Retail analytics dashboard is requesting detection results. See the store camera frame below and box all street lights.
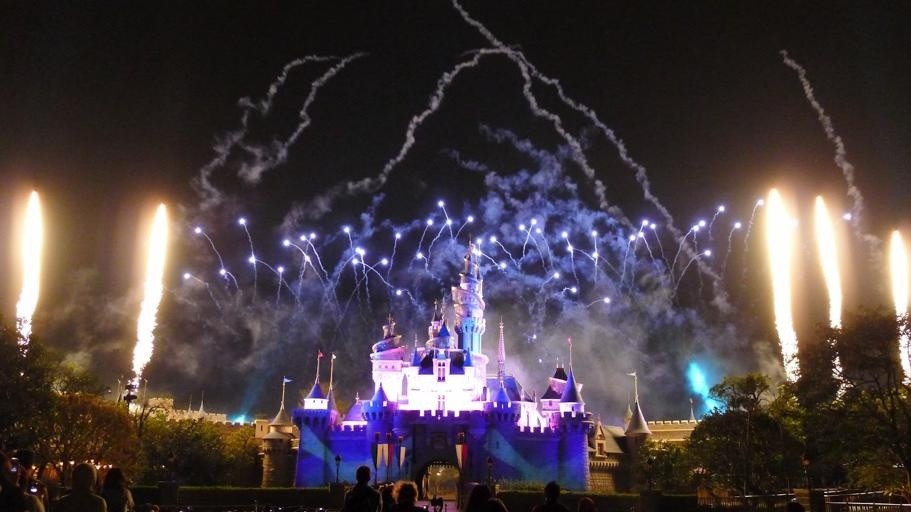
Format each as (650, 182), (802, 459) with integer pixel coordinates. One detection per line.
(334, 453), (341, 483)
(487, 456), (493, 483)
(454, 431), (469, 471)
(383, 432), (395, 482)
(372, 432), (382, 487)
(396, 436), (406, 479)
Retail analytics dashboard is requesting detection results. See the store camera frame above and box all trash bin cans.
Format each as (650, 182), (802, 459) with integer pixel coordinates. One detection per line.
(156, 481), (179, 506)
(809, 489), (826, 512)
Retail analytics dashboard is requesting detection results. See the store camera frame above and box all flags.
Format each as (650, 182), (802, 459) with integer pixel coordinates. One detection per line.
(318, 352), (323, 358)
(332, 353), (336, 360)
(284, 378), (293, 383)
(626, 372), (637, 377)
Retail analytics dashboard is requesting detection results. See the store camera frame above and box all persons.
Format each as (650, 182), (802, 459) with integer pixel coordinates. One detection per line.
(0, 448), (160, 512)
(337, 463), (596, 512)
